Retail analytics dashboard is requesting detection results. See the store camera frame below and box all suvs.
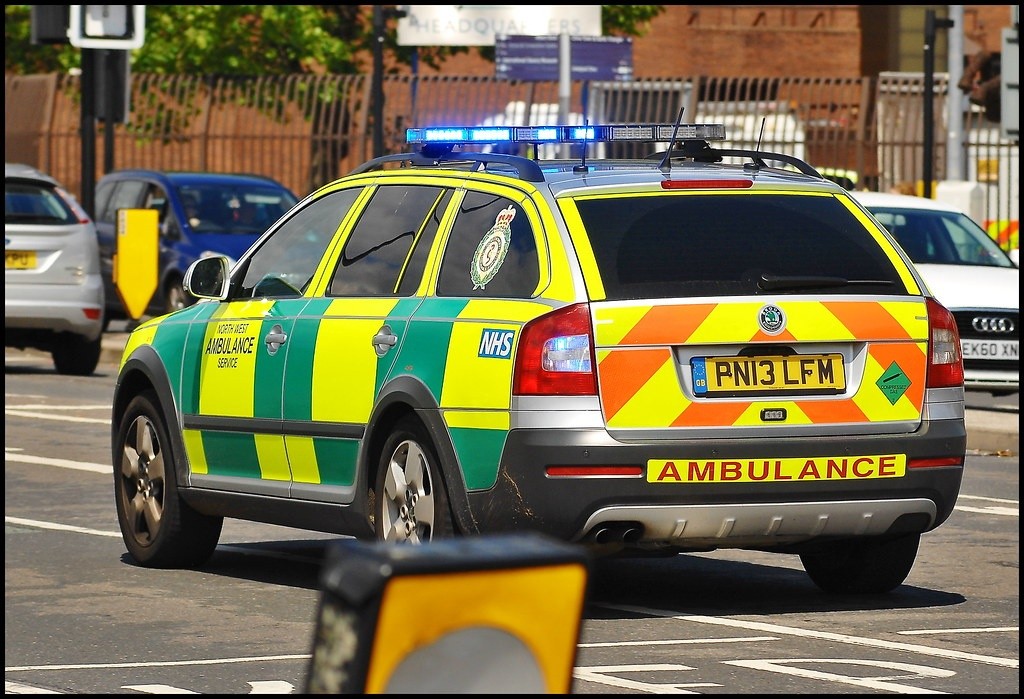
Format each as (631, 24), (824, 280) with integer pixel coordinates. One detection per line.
(111, 123), (968, 598)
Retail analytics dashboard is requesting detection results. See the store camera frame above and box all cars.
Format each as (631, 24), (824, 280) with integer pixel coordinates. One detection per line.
(843, 188), (1019, 401)
(4, 160), (108, 378)
(92, 168), (328, 335)
